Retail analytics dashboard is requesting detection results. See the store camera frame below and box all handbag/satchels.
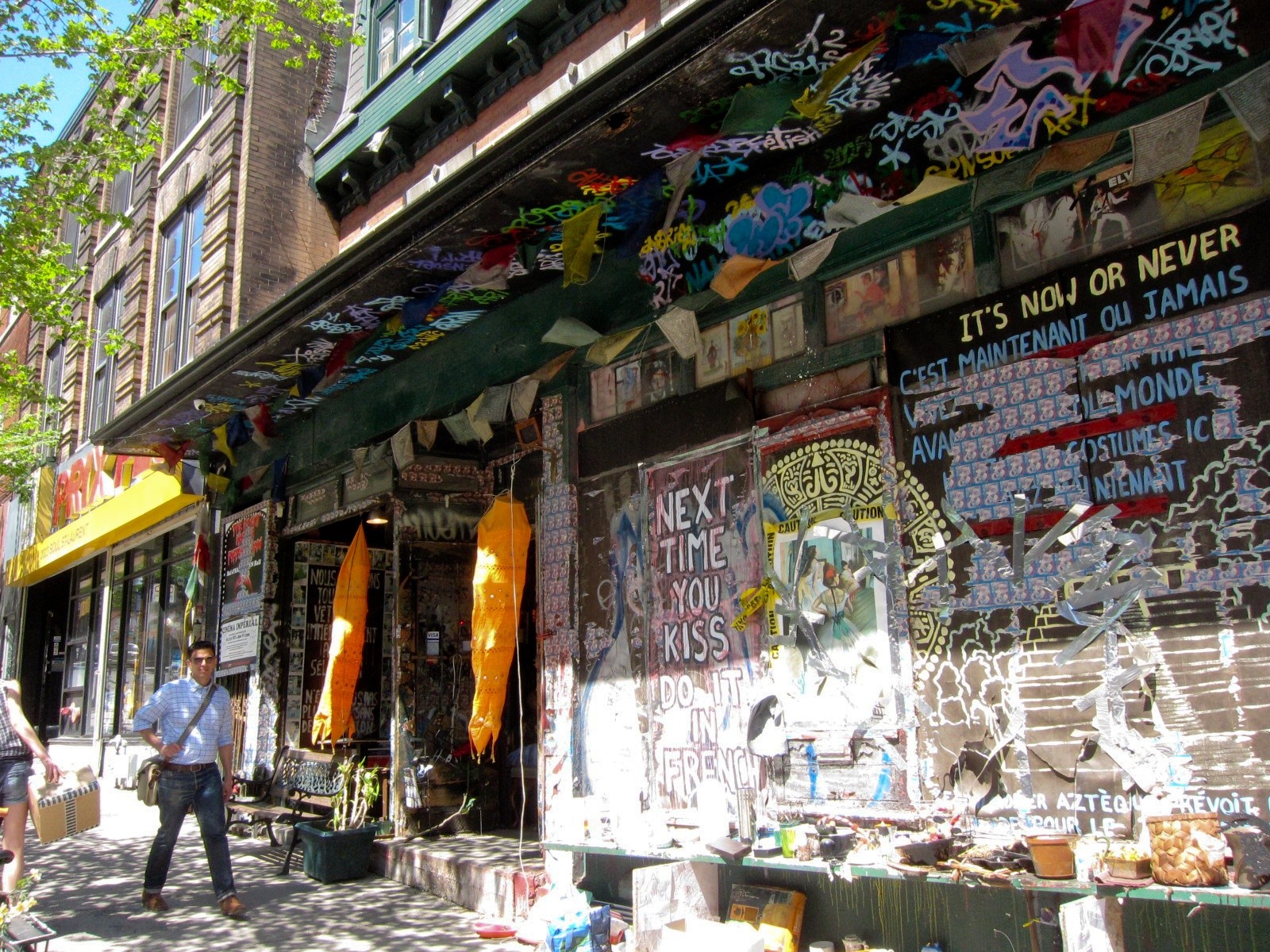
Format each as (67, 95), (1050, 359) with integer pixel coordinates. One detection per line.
(138, 757), (163, 806)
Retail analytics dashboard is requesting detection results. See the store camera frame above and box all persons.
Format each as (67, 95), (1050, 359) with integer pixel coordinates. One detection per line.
(0, 677), (68, 908)
(133, 641), (250, 919)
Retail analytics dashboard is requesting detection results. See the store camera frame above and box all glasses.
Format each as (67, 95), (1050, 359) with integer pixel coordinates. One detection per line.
(189, 657), (215, 663)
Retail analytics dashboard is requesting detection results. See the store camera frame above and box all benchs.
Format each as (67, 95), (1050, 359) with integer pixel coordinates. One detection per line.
(539, 832), (1269, 951)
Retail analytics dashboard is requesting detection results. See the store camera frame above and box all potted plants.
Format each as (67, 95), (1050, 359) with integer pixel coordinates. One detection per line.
(293, 755), (381, 885)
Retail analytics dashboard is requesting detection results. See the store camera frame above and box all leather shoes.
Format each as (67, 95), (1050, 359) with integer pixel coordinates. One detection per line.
(142, 890), (168, 911)
(219, 896), (247, 916)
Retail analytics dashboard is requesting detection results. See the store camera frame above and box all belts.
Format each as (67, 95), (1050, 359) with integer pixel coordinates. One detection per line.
(158, 761), (217, 772)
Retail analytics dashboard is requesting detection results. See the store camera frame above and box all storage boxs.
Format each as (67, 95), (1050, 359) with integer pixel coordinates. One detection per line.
(28, 763), (102, 846)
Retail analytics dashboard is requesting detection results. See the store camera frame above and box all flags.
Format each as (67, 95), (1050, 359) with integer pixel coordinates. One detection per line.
(151, 0), (1125, 666)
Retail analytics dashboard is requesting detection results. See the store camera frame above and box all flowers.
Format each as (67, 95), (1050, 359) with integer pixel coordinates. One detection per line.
(0, 867), (43, 944)
